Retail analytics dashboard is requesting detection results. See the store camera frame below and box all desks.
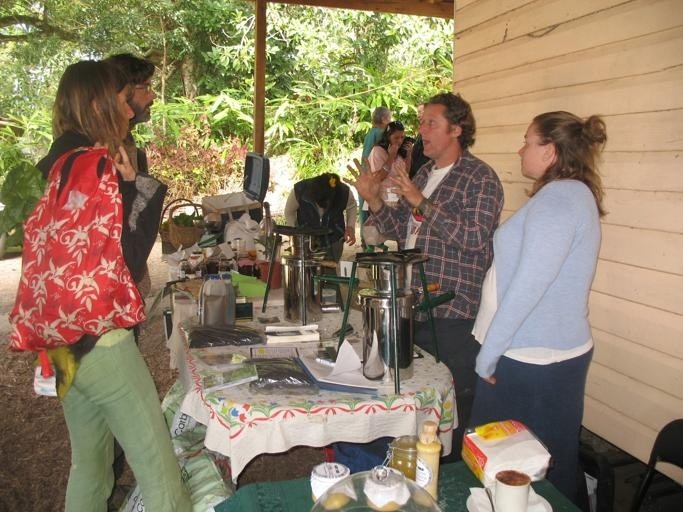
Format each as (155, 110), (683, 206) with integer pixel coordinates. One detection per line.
(176, 294), (458, 477)
(215, 462), (577, 510)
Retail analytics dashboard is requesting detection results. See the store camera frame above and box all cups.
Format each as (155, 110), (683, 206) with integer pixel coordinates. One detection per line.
(494, 467), (532, 511)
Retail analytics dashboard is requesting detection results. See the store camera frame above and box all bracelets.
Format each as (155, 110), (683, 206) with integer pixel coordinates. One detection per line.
(381, 162), (393, 175)
(416, 198), (427, 215)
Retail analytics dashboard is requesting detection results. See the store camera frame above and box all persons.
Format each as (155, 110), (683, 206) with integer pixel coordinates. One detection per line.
(407, 102), (432, 180)
(97, 52), (148, 480)
(359, 119), (412, 253)
(340, 93), (507, 461)
(6, 61), (185, 512)
(359, 107), (392, 226)
(285, 172), (357, 271)
(459, 111), (608, 512)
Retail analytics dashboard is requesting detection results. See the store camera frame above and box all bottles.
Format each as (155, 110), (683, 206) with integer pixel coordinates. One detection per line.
(241, 251), (262, 279)
(415, 422), (442, 509)
(389, 434), (416, 480)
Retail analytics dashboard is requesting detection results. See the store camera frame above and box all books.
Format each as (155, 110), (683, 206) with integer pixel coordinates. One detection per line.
(202, 364), (258, 393)
(299, 340), (378, 390)
(293, 357), (376, 394)
(264, 324), (319, 345)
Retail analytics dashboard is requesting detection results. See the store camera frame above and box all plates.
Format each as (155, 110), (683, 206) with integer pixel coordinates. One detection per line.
(464, 486), (553, 511)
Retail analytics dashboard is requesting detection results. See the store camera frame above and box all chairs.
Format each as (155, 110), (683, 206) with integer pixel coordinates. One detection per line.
(627, 417), (683, 512)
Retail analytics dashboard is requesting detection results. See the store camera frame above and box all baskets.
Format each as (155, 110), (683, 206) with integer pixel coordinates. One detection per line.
(158, 197), (209, 255)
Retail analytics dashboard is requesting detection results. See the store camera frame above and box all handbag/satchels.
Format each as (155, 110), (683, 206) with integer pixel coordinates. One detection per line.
(6, 147), (149, 352)
